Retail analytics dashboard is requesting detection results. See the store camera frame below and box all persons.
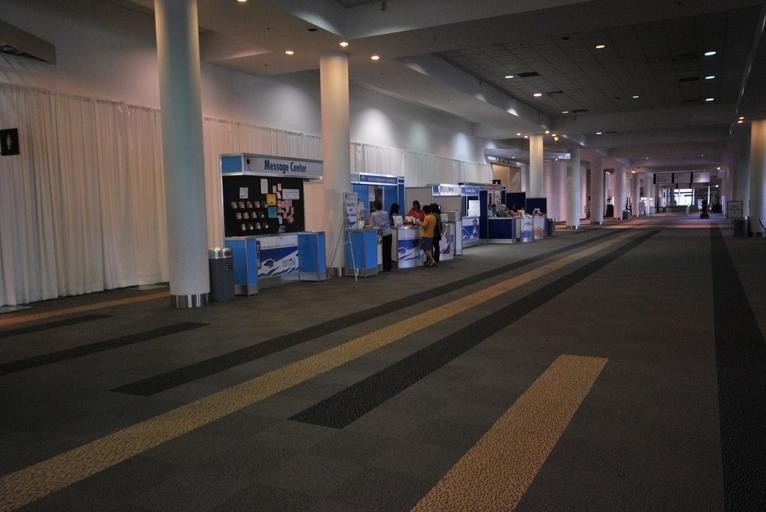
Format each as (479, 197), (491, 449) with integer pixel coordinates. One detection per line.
(369, 200), (443, 273)
(701, 197), (707, 214)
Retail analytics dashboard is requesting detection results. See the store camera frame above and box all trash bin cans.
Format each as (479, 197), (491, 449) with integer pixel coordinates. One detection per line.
(734, 215), (749, 236)
(623, 209), (630, 220)
(208, 247), (236, 303)
(547, 217), (555, 237)
